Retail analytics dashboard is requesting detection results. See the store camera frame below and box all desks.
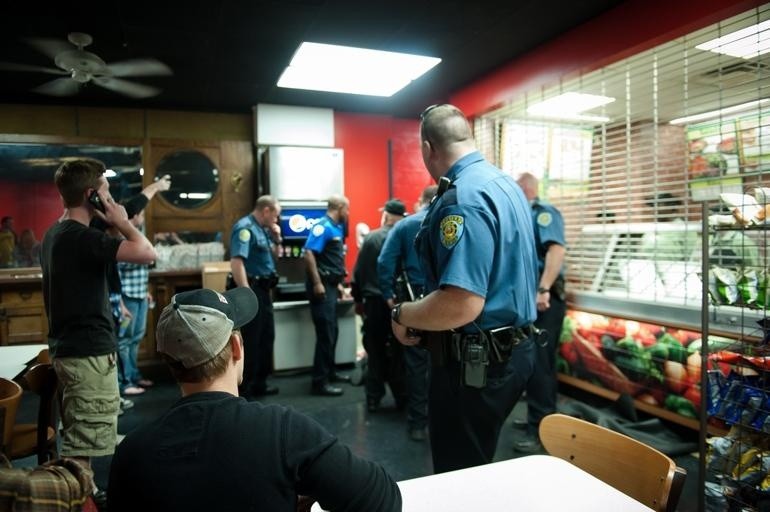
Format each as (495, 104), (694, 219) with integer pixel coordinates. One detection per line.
(0, 344), (49, 382)
(311, 454), (658, 512)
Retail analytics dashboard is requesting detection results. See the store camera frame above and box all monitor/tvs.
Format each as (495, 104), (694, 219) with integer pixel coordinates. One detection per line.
(276, 202), (327, 239)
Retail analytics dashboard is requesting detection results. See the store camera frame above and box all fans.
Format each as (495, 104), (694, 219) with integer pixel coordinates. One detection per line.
(3, 32), (172, 100)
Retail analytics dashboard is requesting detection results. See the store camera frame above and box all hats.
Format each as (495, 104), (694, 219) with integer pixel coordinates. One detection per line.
(378, 199), (407, 218)
(156, 286), (259, 376)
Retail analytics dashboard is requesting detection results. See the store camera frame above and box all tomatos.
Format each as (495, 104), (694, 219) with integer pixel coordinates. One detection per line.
(560, 309), (762, 427)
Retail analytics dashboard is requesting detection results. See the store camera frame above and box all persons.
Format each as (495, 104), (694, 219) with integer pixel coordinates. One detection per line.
(637, 192), (706, 263)
(352, 172), (570, 456)
(39, 157), (173, 499)
(303, 192), (351, 396)
(593, 208), (617, 224)
(0, 455), (95, 512)
(0, 216), (42, 268)
(224, 194), (284, 404)
(106, 287), (403, 511)
(389, 101), (537, 476)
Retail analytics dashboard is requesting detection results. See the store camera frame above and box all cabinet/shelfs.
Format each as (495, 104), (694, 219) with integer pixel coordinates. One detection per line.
(693, 185), (770, 512)
(558, 223), (770, 436)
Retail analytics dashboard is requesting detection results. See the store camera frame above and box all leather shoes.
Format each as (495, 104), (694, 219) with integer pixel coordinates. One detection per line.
(512, 434), (544, 454)
(513, 418), (529, 431)
(366, 399), (379, 410)
(251, 385), (278, 397)
(410, 423), (427, 441)
(309, 383), (344, 396)
(327, 372), (351, 383)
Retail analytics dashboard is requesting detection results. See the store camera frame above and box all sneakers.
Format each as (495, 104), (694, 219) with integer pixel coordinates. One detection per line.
(136, 379), (153, 386)
(118, 397), (134, 408)
(121, 386), (145, 394)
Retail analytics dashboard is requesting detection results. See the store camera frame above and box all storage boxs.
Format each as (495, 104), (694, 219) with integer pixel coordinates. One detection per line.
(201, 261), (232, 292)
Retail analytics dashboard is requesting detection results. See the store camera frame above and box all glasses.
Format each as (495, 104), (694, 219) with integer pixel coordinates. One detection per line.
(420, 104), (440, 152)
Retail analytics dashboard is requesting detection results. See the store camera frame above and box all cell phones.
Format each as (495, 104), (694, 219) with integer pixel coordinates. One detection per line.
(89, 190), (105, 214)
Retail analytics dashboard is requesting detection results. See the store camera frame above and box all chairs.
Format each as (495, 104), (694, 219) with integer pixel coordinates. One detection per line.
(538, 412), (687, 512)
(0, 349), (61, 466)
